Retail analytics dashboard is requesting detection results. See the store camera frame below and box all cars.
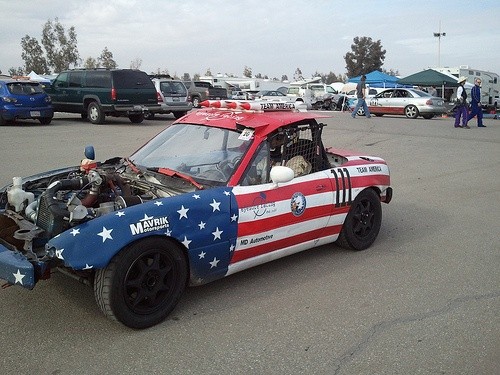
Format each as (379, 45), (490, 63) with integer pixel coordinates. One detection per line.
(350, 88), (449, 119)
(0, 76), (55, 125)
(232, 84), (384, 111)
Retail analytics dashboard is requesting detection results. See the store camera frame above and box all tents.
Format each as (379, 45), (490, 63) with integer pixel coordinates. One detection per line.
(397, 69), (458, 87)
(349, 70), (399, 82)
(17, 71), (51, 84)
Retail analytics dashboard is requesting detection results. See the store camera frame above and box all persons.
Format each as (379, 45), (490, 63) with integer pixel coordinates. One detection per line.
(242, 124), (312, 189)
(453, 77), (471, 129)
(467, 78), (486, 127)
(351, 75), (371, 118)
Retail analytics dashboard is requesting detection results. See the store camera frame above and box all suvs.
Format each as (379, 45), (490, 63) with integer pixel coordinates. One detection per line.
(143, 74), (193, 120)
(41, 67), (161, 124)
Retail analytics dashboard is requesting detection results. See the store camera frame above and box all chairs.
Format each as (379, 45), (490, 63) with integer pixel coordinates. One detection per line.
(13, 85), (22, 93)
(23, 86), (31, 93)
(175, 84), (182, 91)
(281, 139), (324, 176)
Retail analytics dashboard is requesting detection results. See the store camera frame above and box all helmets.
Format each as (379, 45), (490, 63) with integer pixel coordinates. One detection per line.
(267, 124), (300, 158)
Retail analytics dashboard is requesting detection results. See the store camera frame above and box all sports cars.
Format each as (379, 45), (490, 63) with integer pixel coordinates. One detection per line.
(0, 98), (393, 331)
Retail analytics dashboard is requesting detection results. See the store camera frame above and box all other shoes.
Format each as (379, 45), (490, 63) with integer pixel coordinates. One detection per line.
(366, 116), (371, 118)
(351, 115), (356, 118)
(455, 125), (463, 128)
(462, 125), (470, 129)
(477, 124), (486, 127)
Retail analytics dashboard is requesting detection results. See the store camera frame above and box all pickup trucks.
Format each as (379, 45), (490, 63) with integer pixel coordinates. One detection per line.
(185, 81), (228, 109)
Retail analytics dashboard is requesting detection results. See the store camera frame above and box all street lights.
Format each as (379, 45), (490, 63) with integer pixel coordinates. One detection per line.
(433, 23), (447, 67)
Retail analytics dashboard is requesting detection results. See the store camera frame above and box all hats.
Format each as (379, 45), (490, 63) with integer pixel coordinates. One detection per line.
(457, 76), (468, 83)
